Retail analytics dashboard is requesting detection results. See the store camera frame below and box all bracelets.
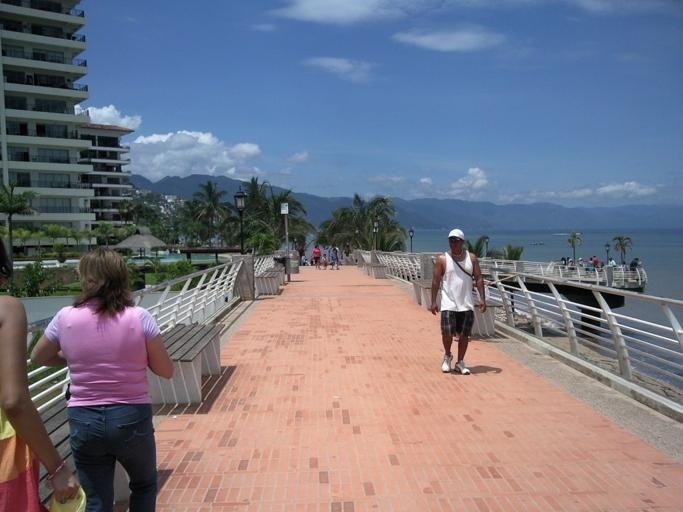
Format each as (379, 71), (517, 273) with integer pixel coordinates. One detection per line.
(46, 460), (66, 479)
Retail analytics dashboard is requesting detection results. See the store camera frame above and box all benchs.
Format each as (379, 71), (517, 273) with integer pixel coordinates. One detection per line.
(254, 270), (282, 296)
(364, 261), (388, 279)
(263, 265), (287, 287)
(148, 321), (226, 405)
(470, 295), (504, 339)
(412, 278), (442, 314)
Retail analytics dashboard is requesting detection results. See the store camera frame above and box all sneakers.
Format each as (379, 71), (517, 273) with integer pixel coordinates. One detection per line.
(455, 361), (470, 375)
(440, 354), (452, 372)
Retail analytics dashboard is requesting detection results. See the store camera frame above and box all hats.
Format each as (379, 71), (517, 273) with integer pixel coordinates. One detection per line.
(448, 228), (464, 239)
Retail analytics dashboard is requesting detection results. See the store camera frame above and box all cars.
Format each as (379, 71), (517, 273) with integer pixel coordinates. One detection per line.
(11, 258), (82, 271)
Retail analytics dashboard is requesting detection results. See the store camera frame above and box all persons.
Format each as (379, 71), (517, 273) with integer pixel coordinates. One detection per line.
(561, 255), (640, 271)
(301, 242), (351, 270)
(430, 229), (486, 375)
(31, 247), (175, 512)
(0, 239), (79, 512)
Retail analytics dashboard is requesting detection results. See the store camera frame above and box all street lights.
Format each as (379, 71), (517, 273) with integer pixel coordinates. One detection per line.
(293, 236), (296, 249)
(408, 225), (414, 253)
(484, 236), (490, 253)
(234, 184), (247, 255)
(604, 241), (611, 265)
(371, 215), (379, 250)
(354, 228), (360, 249)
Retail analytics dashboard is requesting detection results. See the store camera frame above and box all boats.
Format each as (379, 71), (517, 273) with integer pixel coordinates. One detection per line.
(528, 241), (544, 245)
(551, 232), (582, 236)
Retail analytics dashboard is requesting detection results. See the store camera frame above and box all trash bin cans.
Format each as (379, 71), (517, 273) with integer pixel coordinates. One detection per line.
(273, 257), (287, 275)
